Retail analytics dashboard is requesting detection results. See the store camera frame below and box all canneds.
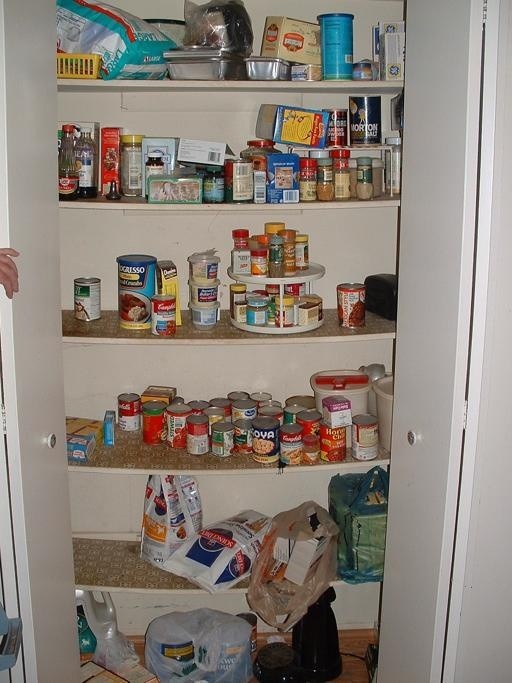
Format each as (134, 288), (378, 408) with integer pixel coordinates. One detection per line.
(116, 254), (156, 327)
(151, 295), (176, 338)
(118, 392), (380, 467)
(337, 282), (367, 329)
(348, 93), (382, 147)
(224, 158), (254, 203)
(230, 282), (306, 328)
(322, 108), (347, 148)
(74, 277), (101, 321)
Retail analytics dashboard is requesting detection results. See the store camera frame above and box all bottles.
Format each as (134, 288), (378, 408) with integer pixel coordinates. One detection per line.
(56, 122), (95, 200)
(119, 132), (144, 199)
(229, 221), (312, 278)
(241, 139), (279, 161)
(299, 148), (384, 200)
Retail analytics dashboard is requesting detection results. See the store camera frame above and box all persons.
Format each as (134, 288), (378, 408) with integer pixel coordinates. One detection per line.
(0, 247), (21, 300)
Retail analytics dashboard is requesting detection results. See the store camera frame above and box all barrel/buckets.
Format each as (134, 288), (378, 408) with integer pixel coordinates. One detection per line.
(308, 369), (372, 418)
(76, 590), (119, 665)
(372, 375), (394, 454)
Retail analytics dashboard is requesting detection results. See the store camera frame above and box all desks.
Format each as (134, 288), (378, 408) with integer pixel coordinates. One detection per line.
(80, 630), (377, 680)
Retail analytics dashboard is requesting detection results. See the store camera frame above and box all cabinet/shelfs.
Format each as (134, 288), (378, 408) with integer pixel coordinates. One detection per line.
(49, 62), (395, 606)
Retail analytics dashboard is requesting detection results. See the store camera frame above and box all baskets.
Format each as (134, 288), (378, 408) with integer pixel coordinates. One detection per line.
(56, 53), (102, 78)
